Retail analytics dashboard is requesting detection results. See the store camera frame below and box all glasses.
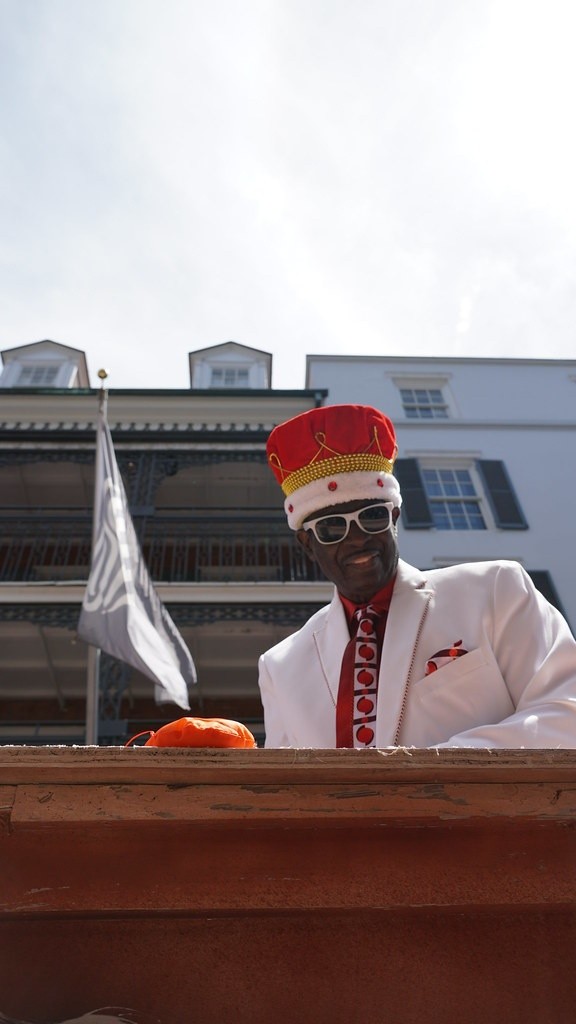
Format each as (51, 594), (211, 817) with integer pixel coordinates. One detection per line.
(303, 502), (392, 545)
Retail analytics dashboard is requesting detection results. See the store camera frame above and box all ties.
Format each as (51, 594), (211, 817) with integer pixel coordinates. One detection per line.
(336, 607), (381, 748)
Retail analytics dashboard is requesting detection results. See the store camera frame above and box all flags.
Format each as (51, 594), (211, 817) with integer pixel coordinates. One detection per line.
(75, 411), (200, 712)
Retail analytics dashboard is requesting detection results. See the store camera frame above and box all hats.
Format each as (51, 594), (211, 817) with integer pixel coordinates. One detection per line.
(265, 404), (402, 531)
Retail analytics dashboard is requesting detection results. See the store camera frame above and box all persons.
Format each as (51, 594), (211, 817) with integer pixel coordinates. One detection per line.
(255, 404), (576, 752)
